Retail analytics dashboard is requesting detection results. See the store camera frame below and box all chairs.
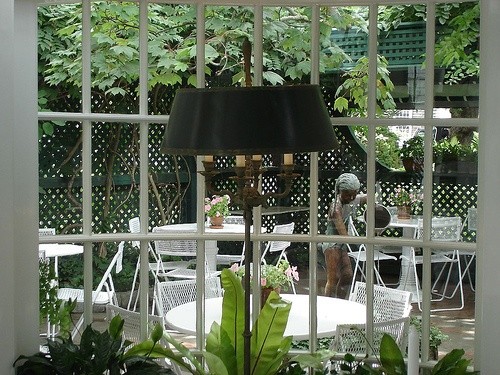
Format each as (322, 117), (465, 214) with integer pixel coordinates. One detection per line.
(105, 277), (413, 370)
(127, 217), (296, 311)
(39, 228), (124, 342)
(346, 208), (477, 313)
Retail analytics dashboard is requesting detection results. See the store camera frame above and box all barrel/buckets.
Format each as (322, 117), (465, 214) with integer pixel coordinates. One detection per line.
(364, 204), (393, 235)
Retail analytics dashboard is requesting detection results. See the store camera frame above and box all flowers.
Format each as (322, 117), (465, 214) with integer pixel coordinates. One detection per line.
(390, 188), (417, 206)
(205, 195), (231, 217)
(230, 258), (299, 291)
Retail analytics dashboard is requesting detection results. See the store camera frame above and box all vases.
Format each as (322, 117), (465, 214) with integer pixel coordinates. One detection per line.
(263, 289), (280, 308)
(397, 204), (411, 219)
(211, 216), (225, 226)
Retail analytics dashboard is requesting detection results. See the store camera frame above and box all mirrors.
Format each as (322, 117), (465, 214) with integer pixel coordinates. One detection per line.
(364, 204), (392, 229)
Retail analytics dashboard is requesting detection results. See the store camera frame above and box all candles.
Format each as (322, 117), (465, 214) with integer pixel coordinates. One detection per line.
(284, 154), (293, 165)
(236, 155), (245, 167)
(204, 155), (213, 162)
(253, 155), (262, 160)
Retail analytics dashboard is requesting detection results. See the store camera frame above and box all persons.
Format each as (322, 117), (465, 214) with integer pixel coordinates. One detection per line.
(322, 173), (367, 299)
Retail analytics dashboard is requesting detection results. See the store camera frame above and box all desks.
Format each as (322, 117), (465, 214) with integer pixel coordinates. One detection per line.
(166, 293), (367, 341)
(38, 244), (84, 339)
(156, 223), (267, 271)
(229, 206), (309, 215)
(356, 215), (457, 304)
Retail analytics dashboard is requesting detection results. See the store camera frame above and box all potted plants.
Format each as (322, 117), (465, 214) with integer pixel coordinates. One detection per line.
(414, 318), (449, 361)
(394, 135), (478, 174)
